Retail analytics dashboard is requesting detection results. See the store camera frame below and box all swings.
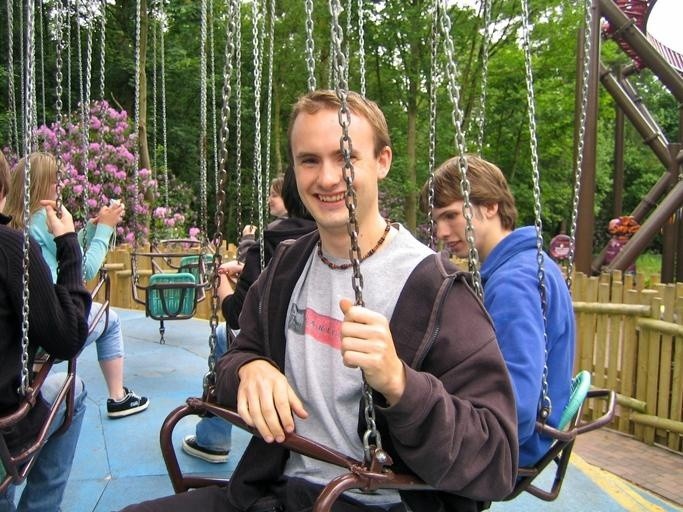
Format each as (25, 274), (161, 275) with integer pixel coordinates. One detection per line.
(0, 3), (615, 512)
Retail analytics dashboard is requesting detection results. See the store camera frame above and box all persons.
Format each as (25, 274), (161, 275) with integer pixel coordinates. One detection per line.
(234, 177), (288, 278)
(602, 214), (640, 286)
(181, 164), (318, 466)
(3, 150), (149, 419)
(0, 149), (87, 510)
(115, 88), (519, 510)
(416, 151), (576, 490)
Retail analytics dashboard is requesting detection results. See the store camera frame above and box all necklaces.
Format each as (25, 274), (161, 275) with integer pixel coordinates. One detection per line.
(314, 220), (393, 270)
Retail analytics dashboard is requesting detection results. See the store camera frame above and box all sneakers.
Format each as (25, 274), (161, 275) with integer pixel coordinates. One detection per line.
(182, 435), (229, 464)
(107, 387), (149, 417)
